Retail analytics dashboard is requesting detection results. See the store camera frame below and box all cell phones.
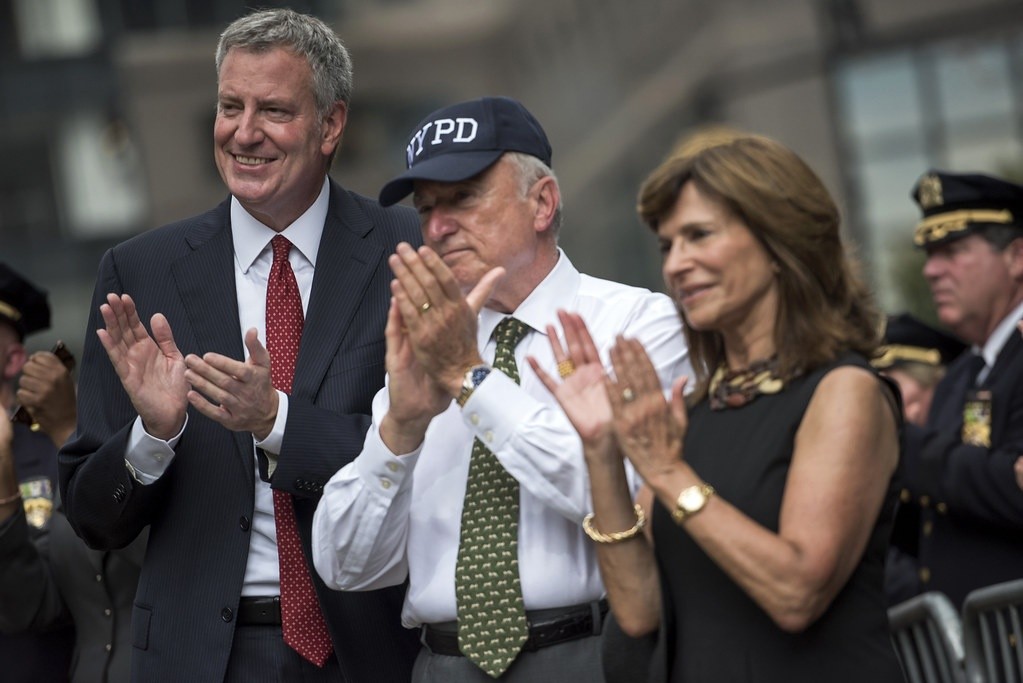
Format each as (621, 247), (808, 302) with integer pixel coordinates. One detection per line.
(10, 340), (75, 428)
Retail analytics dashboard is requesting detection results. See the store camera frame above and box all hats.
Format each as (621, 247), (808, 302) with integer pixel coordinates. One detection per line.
(870, 311), (968, 371)
(379, 98), (552, 209)
(910, 169), (1023, 245)
(0, 264), (52, 331)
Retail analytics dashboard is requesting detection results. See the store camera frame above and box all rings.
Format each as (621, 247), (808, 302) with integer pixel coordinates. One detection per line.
(419, 302), (431, 314)
(621, 386), (635, 403)
(558, 359), (574, 378)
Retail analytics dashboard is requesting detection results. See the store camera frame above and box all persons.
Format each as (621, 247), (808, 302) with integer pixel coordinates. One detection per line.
(311, 96), (697, 683)
(0, 260), (152, 683)
(869, 168), (1023, 683)
(524, 133), (906, 683)
(57, 8), (424, 683)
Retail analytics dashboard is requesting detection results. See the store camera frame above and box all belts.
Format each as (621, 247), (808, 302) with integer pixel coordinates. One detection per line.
(423, 601), (608, 656)
(239, 597), (282, 627)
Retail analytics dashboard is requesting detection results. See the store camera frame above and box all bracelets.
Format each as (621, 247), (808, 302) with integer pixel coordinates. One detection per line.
(0, 489), (23, 504)
(582, 504), (647, 543)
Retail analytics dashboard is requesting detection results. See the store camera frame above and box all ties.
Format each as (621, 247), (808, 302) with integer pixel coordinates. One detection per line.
(270, 235), (333, 666)
(455, 319), (529, 680)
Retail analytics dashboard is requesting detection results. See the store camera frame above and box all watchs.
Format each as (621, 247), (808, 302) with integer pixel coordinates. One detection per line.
(456, 365), (492, 408)
(671, 484), (716, 526)
